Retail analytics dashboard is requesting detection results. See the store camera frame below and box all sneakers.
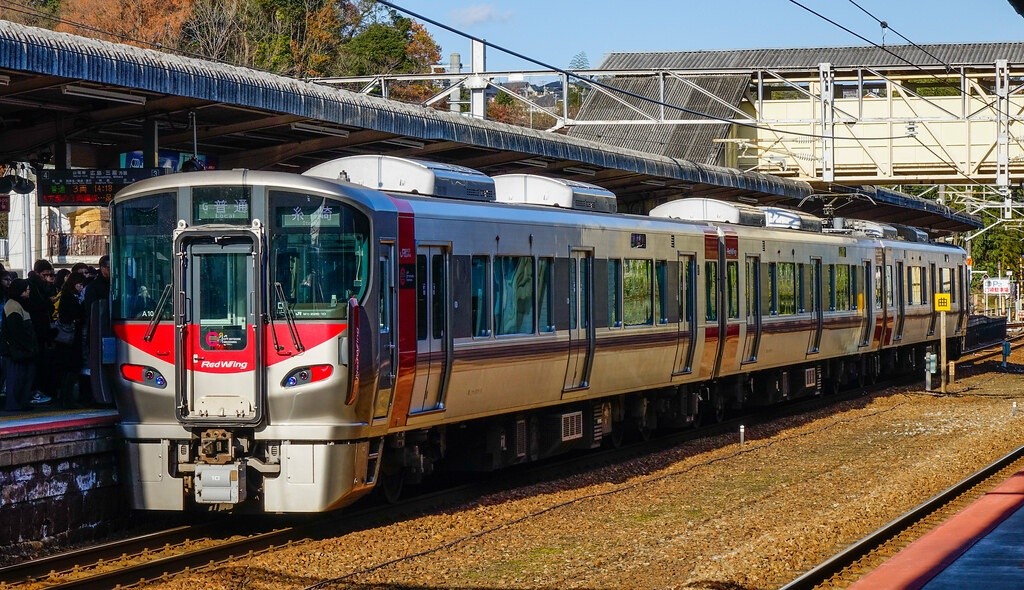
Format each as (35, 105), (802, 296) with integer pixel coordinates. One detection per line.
(29, 391), (52, 403)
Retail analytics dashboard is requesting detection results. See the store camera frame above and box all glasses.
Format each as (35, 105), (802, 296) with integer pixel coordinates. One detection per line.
(42, 273), (54, 277)
(2, 278), (12, 281)
(78, 270), (89, 275)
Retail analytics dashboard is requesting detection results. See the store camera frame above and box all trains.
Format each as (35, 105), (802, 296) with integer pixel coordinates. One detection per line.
(90, 155), (972, 533)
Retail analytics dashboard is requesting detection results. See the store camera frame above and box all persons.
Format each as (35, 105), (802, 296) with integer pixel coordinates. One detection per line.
(1, 255), (158, 412)
